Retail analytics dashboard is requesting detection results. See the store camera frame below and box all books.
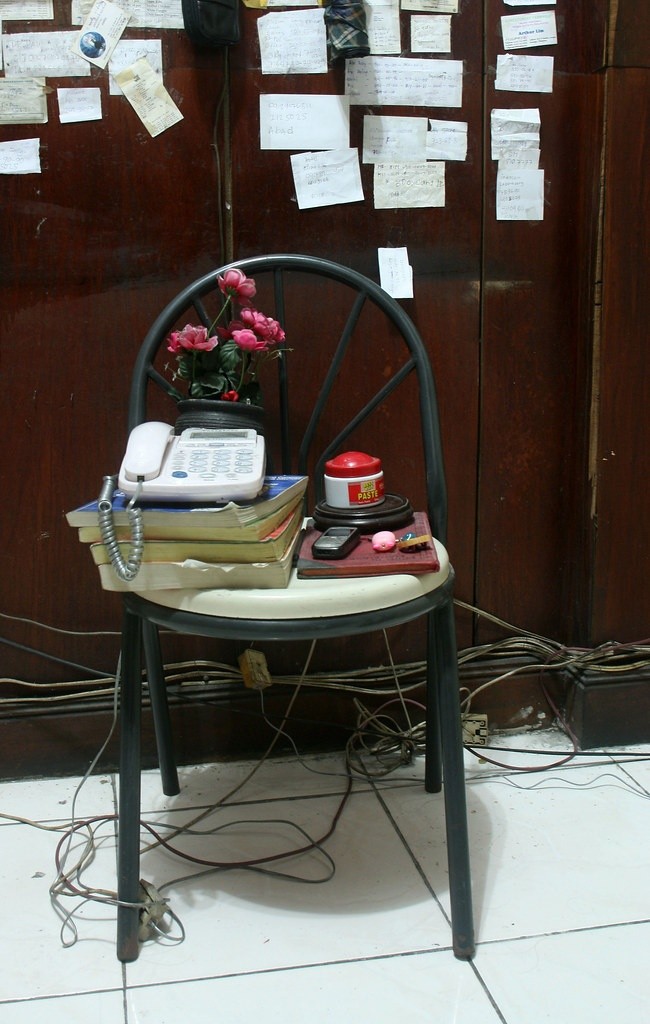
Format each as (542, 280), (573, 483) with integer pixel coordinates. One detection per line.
(296, 512), (441, 579)
(65, 476), (311, 592)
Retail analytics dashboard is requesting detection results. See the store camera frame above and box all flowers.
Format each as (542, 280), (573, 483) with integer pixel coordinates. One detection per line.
(163, 268), (296, 405)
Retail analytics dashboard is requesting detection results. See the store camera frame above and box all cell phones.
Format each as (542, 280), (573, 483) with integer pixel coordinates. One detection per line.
(311, 526), (361, 559)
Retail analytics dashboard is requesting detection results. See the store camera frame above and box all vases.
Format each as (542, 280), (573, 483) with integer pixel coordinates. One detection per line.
(171, 400), (266, 438)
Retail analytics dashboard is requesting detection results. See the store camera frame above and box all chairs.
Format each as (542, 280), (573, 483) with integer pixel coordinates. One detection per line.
(117, 253), (476, 961)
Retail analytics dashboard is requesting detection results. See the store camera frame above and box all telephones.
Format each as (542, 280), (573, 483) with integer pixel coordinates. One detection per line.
(98, 421), (266, 582)
